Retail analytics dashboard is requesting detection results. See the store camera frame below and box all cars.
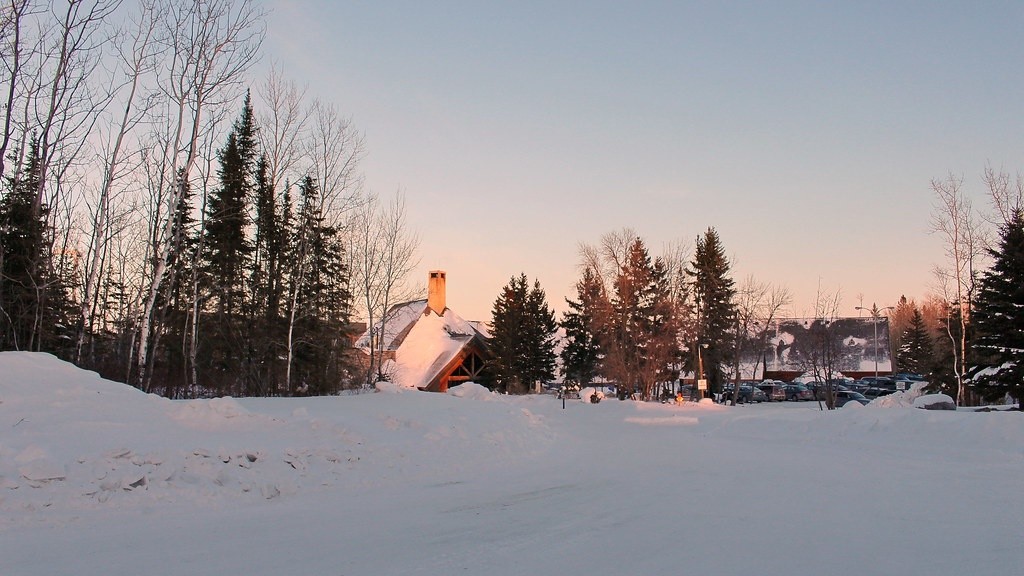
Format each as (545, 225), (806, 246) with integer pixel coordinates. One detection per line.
(825, 390), (872, 408)
(724, 372), (923, 403)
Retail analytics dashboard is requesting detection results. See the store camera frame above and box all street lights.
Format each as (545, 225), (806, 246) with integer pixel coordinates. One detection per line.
(697, 343), (709, 398)
(855, 306), (895, 381)
